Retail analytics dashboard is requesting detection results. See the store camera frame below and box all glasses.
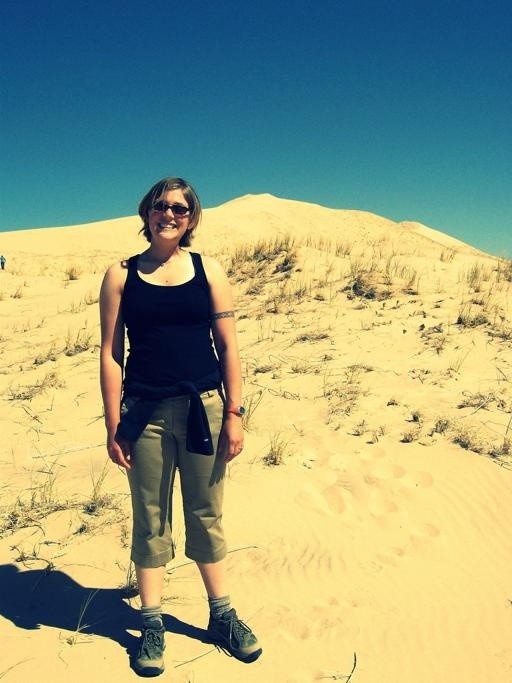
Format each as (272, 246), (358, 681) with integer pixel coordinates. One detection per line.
(152, 200), (192, 214)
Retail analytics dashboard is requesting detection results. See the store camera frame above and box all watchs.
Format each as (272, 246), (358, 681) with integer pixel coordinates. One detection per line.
(225, 405), (245, 415)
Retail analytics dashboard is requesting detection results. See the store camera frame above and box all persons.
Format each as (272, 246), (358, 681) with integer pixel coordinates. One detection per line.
(99, 177), (263, 677)
(0, 255), (6, 270)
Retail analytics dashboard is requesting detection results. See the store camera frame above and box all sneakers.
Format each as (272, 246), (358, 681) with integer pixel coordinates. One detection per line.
(134, 619), (166, 677)
(207, 608), (262, 663)
